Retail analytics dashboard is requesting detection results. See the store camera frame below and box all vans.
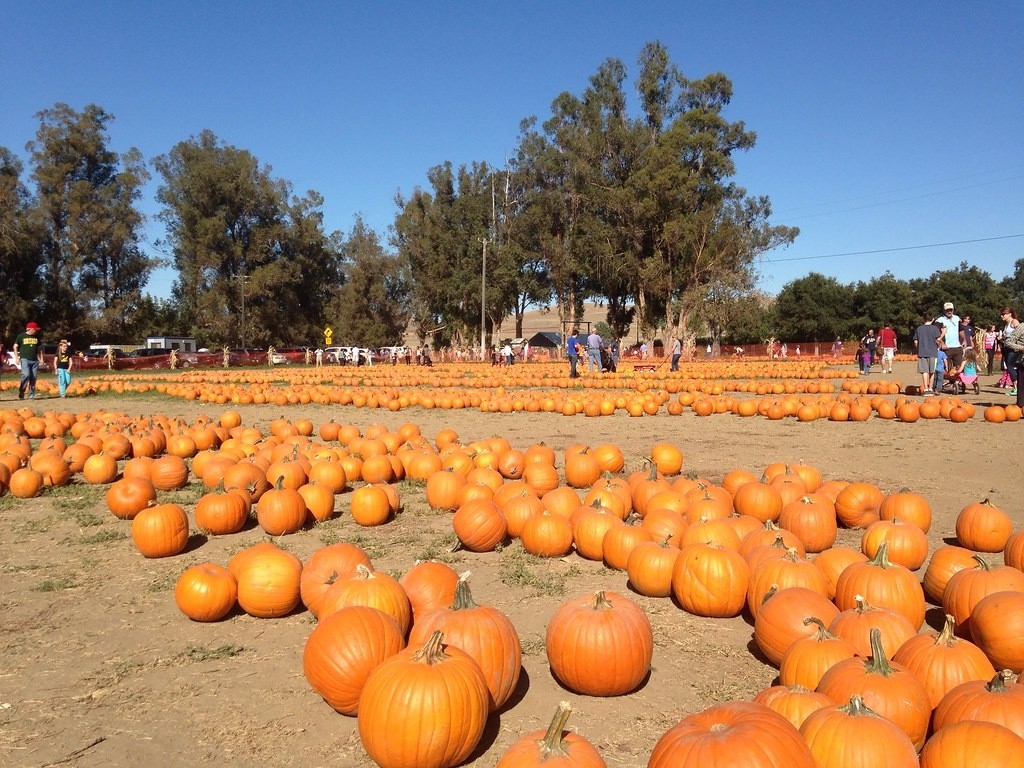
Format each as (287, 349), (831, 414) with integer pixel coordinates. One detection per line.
(305, 347), (406, 363)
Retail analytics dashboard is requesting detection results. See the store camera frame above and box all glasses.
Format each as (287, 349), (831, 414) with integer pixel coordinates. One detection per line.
(1001, 313), (1009, 315)
(672, 337), (674, 338)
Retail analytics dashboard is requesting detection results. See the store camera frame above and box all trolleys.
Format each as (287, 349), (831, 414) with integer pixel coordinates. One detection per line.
(944, 369), (980, 395)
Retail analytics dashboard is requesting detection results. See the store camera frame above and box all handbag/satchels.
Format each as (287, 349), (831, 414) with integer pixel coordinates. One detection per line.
(422, 350), (424, 355)
(1009, 351), (1024, 369)
(875, 345), (883, 356)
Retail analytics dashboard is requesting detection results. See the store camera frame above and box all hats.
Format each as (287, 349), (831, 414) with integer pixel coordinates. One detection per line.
(943, 302), (954, 310)
(27, 322), (41, 330)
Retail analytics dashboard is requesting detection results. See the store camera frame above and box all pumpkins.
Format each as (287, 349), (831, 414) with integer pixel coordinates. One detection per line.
(0, 408), (1024, 768)
(0, 354), (1024, 422)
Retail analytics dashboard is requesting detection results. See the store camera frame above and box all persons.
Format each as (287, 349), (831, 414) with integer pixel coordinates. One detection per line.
(913, 302), (1024, 408)
(640, 342), (647, 360)
(450, 348), (473, 361)
(796, 345), (800, 356)
(856, 329), (877, 375)
(116, 351), (124, 358)
(314, 344), (431, 367)
(13, 322), (43, 399)
(567, 329), (620, 373)
(54, 341), (73, 398)
(79, 350), (84, 357)
(833, 336), (845, 359)
(141, 349), (148, 356)
(733, 346), (744, 359)
(875, 320), (897, 374)
(780, 343), (788, 359)
(670, 337), (681, 372)
(489, 340), (533, 367)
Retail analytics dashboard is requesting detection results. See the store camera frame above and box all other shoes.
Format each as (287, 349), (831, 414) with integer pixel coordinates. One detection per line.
(1005, 389), (1017, 396)
(888, 368), (892, 373)
(923, 391), (934, 396)
(19, 391), (24, 398)
(882, 370), (886, 373)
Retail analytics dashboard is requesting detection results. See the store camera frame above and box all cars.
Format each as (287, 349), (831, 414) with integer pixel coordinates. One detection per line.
(5, 345), (305, 371)
